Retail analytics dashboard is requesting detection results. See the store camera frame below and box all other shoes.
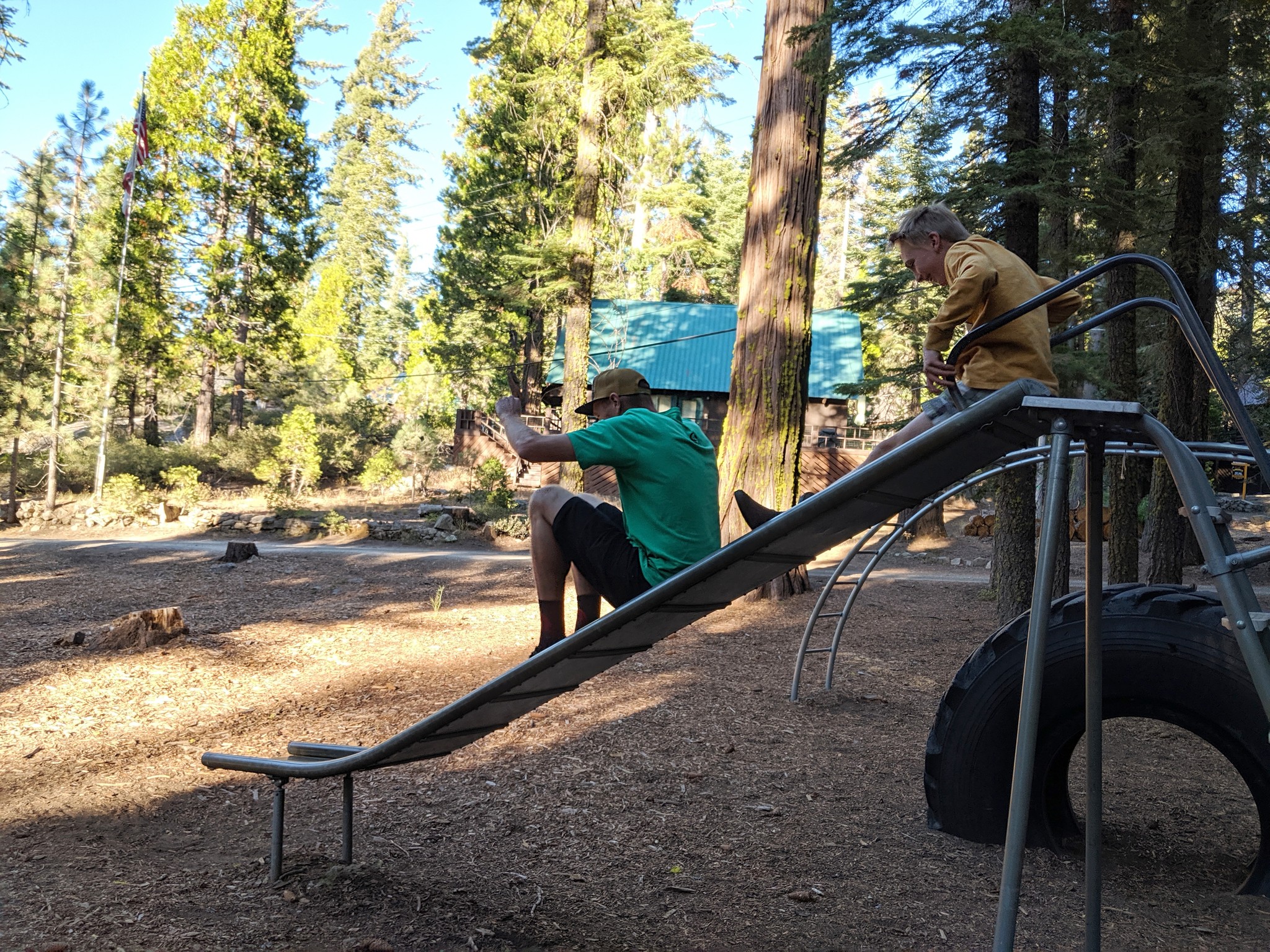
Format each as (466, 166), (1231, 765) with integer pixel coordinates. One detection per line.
(734, 489), (789, 532)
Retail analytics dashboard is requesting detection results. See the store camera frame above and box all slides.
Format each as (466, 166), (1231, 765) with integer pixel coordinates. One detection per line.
(202, 377), (1066, 777)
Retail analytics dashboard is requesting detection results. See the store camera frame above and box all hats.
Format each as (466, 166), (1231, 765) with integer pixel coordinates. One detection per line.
(575, 367), (651, 416)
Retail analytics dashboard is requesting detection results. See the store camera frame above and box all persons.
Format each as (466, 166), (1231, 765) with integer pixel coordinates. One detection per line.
(494, 368), (719, 660)
(734, 203), (1083, 532)
